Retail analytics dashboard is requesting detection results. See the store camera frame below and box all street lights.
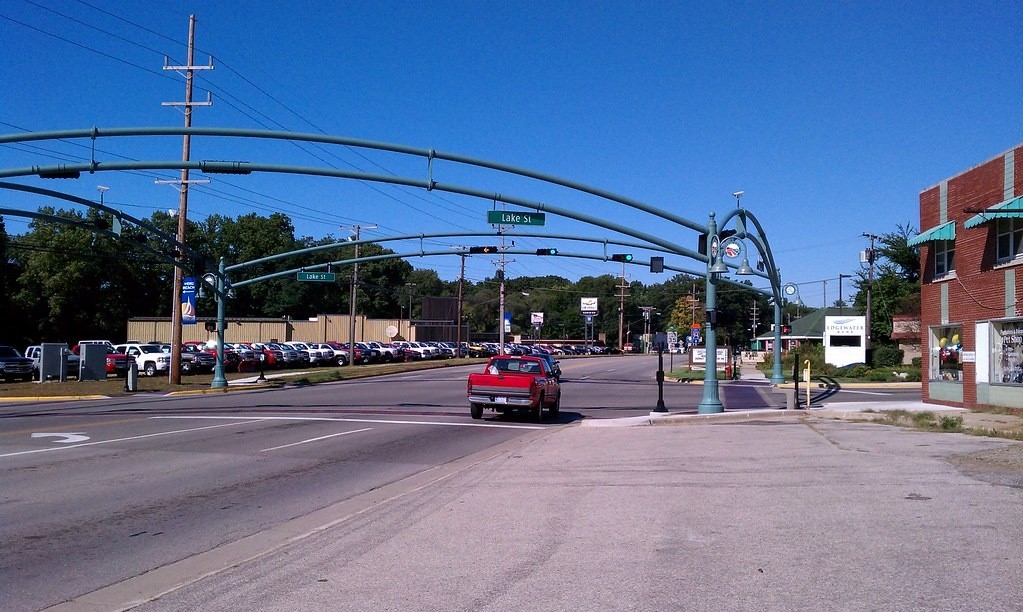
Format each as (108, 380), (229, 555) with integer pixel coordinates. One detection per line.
(698, 212), (789, 413)
(195, 254), (237, 389)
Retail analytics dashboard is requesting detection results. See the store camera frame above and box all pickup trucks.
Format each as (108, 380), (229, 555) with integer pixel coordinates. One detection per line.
(467, 353), (562, 423)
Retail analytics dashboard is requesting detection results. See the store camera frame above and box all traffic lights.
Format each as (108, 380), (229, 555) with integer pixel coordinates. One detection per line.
(536, 248), (557, 256)
(612, 254), (633, 261)
(470, 246), (498, 253)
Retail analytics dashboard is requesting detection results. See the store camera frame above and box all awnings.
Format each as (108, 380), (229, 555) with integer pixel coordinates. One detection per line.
(907, 221), (956, 246)
(963, 195), (1023, 228)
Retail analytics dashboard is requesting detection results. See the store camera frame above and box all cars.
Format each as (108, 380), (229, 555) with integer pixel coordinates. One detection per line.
(78, 339), (624, 374)
(26, 344), (80, 376)
(523, 354), (562, 383)
(1, 345), (34, 383)
(114, 345), (171, 377)
(72, 344), (137, 379)
